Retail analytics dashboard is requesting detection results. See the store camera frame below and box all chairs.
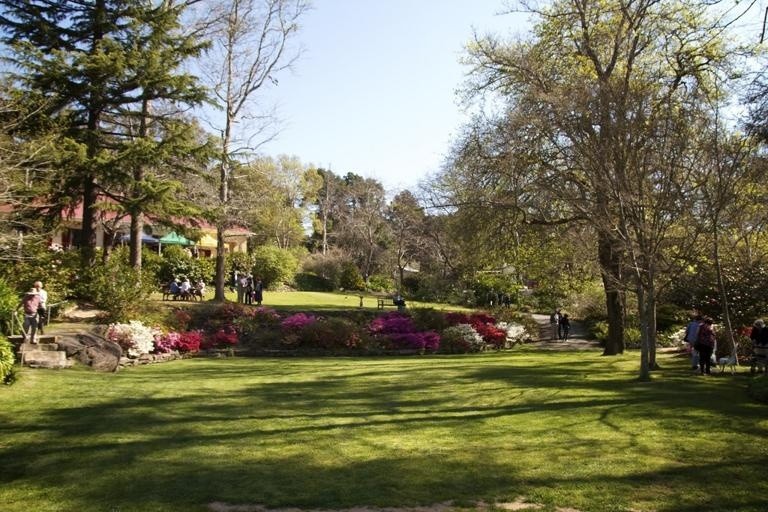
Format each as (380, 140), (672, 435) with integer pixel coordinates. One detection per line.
(718, 343), (740, 375)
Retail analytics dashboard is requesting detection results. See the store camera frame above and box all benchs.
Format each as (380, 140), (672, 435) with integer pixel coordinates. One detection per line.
(377, 293), (399, 311)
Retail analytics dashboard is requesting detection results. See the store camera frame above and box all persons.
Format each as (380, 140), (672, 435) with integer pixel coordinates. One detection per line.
(169, 275), (204, 302)
(550, 308), (571, 341)
(750, 320), (768, 372)
(14, 288), (43, 344)
(497, 290), (510, 308)
(30, 281), (47, 335)
(233, 272), (263, 306)
(393, 292), (406, 311)
(684, 314), (717, 375)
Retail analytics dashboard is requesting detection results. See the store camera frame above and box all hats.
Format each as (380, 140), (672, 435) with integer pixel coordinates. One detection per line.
(23, 287), (42, 295)
(753, 319), (766, 328)
